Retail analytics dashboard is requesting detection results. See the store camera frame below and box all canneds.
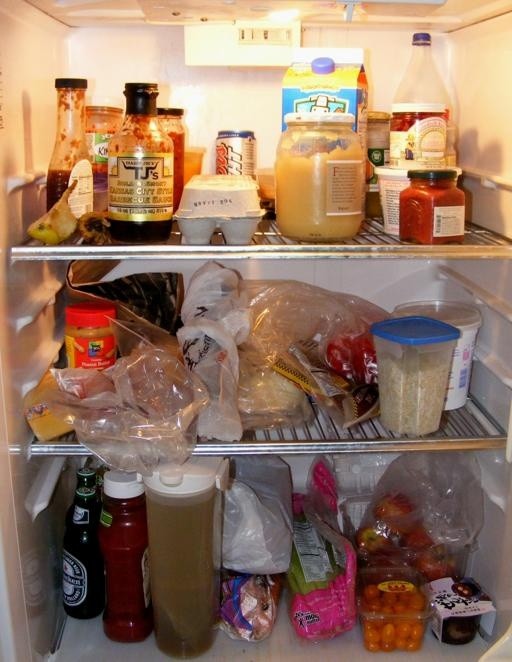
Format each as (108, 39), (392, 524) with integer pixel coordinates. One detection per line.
(214, 129), (256, 178)
(390, 101), (446, 171)
(399, 169), (466, 245)
(64, 300), (118, 372)
(157, 107), (186, 211)
(84, 104), (124, 215)
(366, 108), (391, 217)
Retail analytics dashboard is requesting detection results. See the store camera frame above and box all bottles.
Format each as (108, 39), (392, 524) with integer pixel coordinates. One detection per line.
(16, 520), (47, 618)
(46, 76), (94, 221)
(103, 81), (176, 240)
(100, 472), (153, 642)
(144, 456), (229, 658)
(61, 467), (106, 619)
(390, 33), (452, 168)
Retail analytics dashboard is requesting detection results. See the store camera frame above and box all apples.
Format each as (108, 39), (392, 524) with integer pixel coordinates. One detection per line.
(27, 180), (83, 244)
(354, 492), (455, 584)
